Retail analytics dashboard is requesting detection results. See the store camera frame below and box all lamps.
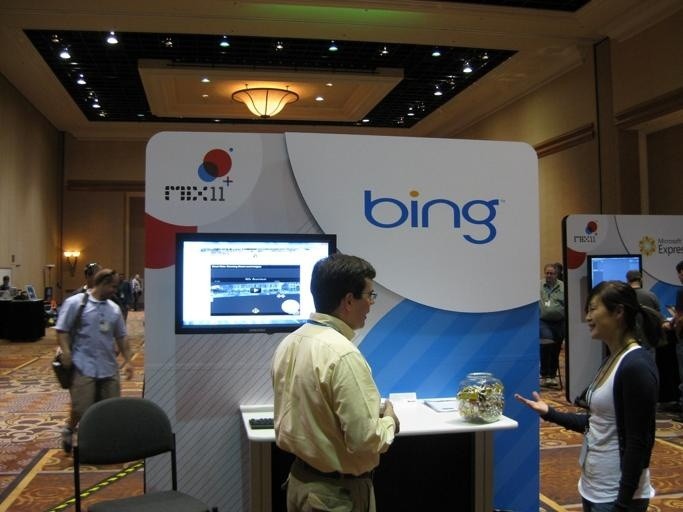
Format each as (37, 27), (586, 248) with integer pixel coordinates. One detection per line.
(64, 252), (80, 265)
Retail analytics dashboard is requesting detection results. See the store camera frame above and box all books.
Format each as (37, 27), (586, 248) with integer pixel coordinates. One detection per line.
(425, 395), (460, 414)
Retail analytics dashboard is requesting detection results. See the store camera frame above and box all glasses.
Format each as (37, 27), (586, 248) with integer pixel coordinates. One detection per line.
(354, 291), (378, 300)
(362, 289), (377, 300)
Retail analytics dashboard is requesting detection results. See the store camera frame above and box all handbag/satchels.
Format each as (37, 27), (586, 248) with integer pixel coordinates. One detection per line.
(52, 353), (75, 390)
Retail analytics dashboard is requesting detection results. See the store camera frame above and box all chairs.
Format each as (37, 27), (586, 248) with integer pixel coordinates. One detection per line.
(74, 397), (219, 512)
(540, 339), (564, 391)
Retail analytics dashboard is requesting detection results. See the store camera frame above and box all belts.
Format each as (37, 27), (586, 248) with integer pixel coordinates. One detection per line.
(299, 462), (374, 480)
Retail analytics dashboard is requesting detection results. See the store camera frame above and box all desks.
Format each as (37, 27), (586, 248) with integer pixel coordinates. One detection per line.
(0, 298), (46, 342)
(240, 398), (519, 512)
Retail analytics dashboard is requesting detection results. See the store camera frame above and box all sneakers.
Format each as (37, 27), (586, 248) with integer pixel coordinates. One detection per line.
(62, 428), (72, 453)
(544, 378), (559, 386)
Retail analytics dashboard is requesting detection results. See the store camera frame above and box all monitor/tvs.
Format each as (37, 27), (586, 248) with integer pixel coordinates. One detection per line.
(587, 253), (644, 293)
(174, 232), (339, 335)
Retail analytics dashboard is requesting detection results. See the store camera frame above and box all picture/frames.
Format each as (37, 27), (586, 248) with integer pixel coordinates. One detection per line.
(0, 268), (12, 291)
(25, 284), (37, 300)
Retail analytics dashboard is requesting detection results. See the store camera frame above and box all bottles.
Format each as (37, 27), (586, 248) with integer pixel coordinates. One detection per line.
(457, 373), (504, 423)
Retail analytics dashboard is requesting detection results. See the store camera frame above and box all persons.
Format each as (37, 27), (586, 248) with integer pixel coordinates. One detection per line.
(54, 267), (136, 460)
(271, 254), (401, 512)
(1, 262), (144, 357)
(553, 262), (566, 284)
(625, 269), (661, 413)
(538, 263), (568, 388)
(661, 260), (683, 422)
(511, 280), (665, 511)
